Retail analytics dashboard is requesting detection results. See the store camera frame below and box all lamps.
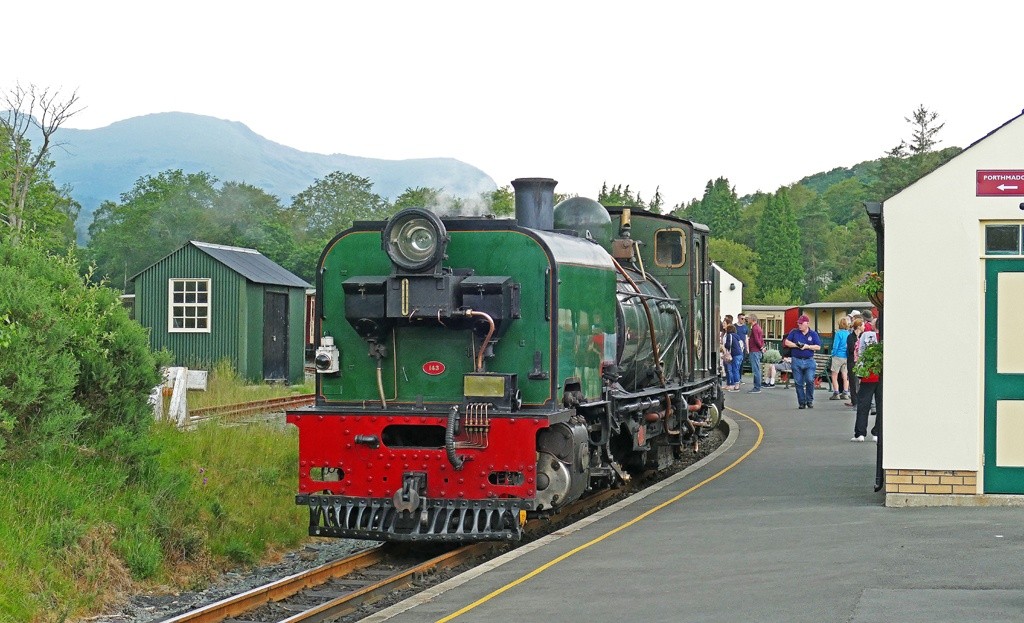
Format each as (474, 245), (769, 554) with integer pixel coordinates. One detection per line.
(822, 309), (827, 312)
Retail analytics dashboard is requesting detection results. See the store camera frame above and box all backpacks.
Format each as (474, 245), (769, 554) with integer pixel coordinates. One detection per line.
(779, 329), (798, 358)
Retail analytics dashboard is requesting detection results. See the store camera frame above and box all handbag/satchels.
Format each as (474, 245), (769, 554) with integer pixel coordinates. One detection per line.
(721, 348), (733, 362)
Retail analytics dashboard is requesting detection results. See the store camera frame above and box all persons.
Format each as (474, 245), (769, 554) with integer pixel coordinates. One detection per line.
(785, 316), (821, 410)
(829, 307), (881, 442)
(718, 313), (766, 393)
(554, 307), (611, 402)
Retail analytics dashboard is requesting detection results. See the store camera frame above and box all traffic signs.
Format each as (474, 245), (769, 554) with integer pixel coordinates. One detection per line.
(977, 170), (1024, 197)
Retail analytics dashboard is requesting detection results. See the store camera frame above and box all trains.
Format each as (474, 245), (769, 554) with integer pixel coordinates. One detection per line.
(275, 177), (724, 542)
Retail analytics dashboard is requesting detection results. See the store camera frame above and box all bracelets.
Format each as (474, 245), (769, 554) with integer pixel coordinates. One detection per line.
(809, 345), (811, 350)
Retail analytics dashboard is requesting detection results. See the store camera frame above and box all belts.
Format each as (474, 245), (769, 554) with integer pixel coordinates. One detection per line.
(796, 357), (810, 359)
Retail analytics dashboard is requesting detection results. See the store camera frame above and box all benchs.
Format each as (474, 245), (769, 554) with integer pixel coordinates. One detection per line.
(783, 353), (832, 392)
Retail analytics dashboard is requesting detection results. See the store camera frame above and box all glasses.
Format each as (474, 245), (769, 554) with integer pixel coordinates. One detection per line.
(741, 318), (744, 320)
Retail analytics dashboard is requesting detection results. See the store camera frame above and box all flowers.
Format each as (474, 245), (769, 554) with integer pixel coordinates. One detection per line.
(853, 269), (884, 296)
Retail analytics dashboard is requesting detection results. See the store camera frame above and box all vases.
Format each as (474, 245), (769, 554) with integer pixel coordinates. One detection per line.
(867, 291), (884, 310)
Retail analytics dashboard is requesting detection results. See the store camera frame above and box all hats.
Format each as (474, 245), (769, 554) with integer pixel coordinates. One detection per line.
(848, 310), (860, 318)
(861, 310), (873, 321)
(870, 307), (878, 318)
(796, 315), (809, 323)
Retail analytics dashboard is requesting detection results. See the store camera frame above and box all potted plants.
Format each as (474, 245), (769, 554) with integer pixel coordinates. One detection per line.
(760, 349), (782, 386)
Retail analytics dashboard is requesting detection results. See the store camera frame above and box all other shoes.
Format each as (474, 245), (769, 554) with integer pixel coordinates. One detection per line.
(872, 436), (877, 441)
(740, 381), (745, 384)
(807, 401), (813, 408)
(799, 403), (805, 409)
(845, 403), (854, 407)
(840, 394), (848, 400)
(722, 385), (734, 389)
(871, 412), (877, 415)
(851, 435), (864, 442)
(728, 387), (740, 393)
(830, 393), (840, 400)
(749, 388), (761, 393)
(761, 382), (775, 388)
